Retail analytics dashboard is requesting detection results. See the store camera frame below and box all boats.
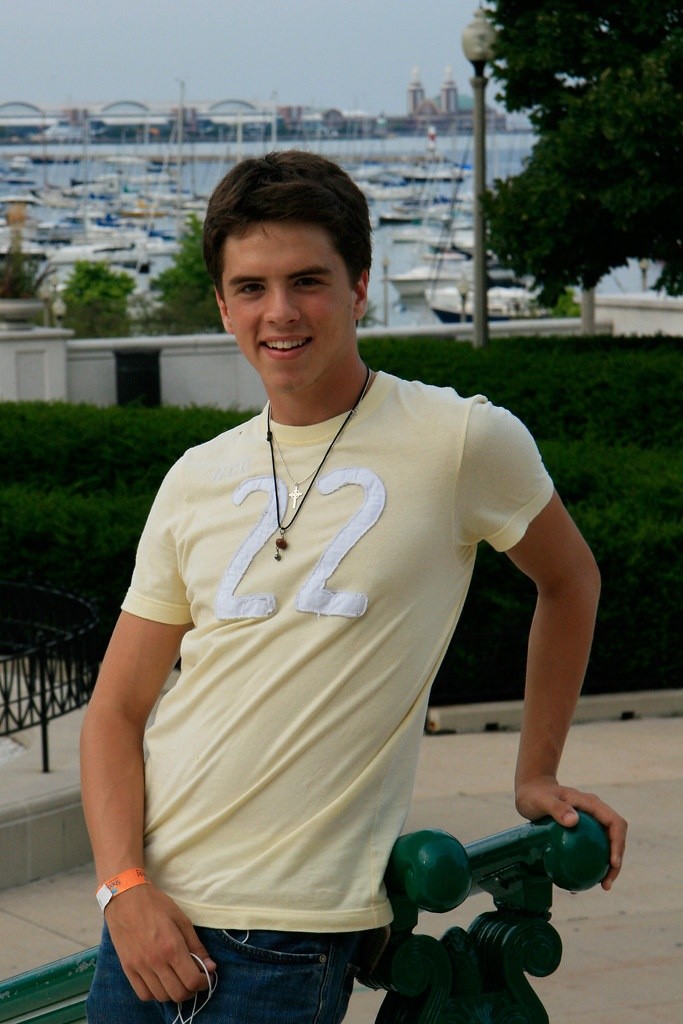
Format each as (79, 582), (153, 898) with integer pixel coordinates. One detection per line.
(4, 64), (559, 324)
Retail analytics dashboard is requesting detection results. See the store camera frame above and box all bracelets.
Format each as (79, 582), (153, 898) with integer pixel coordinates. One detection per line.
(95, 869), (153, 913)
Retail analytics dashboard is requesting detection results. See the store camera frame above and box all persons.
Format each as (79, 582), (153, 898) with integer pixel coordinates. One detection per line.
(78, 151), (627, 1024)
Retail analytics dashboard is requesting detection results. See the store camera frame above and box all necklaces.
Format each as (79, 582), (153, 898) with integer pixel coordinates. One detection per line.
(266, 362), (371, 563)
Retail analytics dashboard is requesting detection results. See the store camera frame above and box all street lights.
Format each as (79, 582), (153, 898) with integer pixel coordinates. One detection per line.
(460, 1), (495, 348)
